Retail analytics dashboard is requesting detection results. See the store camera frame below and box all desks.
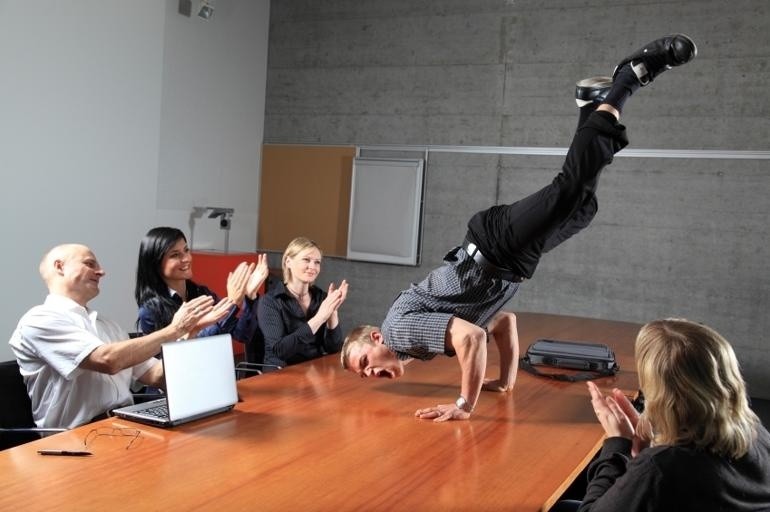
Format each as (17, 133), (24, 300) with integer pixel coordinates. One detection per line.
(0, 313), (644, 512)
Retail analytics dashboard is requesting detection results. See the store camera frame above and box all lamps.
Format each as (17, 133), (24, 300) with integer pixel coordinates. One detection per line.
(179, 0), (215, 21)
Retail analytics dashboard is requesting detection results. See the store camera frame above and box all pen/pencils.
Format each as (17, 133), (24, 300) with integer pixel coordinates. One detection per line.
(37, 450), (94, 456)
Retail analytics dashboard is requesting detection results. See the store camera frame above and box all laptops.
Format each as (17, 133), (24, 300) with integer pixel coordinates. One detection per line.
(111, 333), (239, 429)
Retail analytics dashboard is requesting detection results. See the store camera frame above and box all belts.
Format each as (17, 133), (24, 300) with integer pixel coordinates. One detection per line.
(462, 237), (525, 283)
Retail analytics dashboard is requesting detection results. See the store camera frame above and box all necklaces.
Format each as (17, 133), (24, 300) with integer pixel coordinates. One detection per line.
(289, 286), (308, 301)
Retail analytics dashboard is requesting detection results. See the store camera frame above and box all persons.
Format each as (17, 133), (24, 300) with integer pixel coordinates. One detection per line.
(9, 244), (234, 437)
(258, 236), (350, 372)
(575, 320), (770, 511)
(135, 228), (270, 403)
(341, 33), (697, 422)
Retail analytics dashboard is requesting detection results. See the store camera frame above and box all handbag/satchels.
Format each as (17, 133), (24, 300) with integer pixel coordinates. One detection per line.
(519, 338), (620, 382)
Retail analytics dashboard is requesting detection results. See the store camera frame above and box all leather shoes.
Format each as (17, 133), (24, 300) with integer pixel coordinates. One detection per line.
(613, 33), (698, 87)
(575, 77), (613, 101)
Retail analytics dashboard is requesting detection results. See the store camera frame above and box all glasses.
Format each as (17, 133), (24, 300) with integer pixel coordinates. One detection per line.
(84, 426), (140, 449)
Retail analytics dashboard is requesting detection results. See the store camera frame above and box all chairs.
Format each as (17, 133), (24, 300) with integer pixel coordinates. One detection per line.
(129, 333), (263, 396)
(0, 361), (165, 450)
(237, 329), (283, 370)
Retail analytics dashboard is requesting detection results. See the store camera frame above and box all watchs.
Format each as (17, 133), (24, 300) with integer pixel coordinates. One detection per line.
(456, 398), (475, 414)
